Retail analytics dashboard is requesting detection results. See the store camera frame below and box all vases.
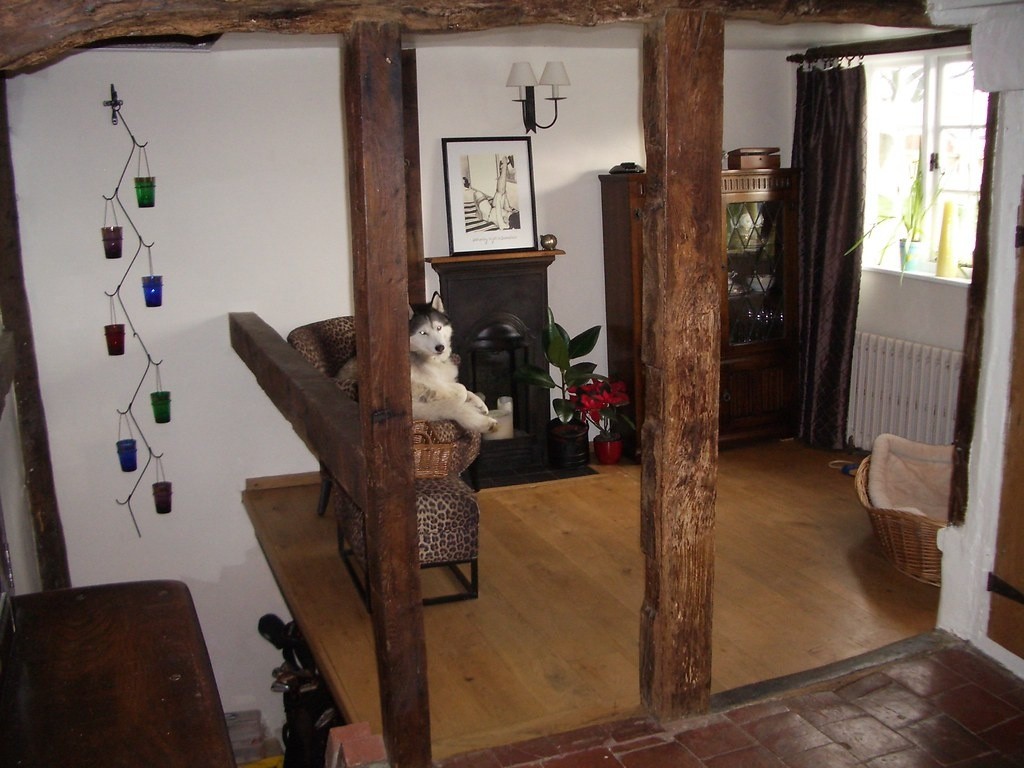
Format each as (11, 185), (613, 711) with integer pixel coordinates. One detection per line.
(593, 431), (623, 465)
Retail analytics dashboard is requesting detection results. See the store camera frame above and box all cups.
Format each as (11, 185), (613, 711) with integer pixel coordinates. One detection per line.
(141, 276), (164, 308)
(150, 390), (171, 423)
(116, 439), (138, 472)
(134, 175), (156, 208)
(153, 481), (172, 513)
(104, 324), (125, 356)
(100, 226), (124, 259)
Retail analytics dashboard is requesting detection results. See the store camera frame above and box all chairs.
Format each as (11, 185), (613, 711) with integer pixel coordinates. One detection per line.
(286, 316), (462, 515)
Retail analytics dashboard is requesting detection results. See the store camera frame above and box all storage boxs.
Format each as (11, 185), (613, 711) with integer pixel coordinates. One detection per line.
(726, 147), (781, 170)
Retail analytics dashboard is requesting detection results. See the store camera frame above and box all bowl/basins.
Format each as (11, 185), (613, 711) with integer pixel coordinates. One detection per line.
(745, 274), (775, 291)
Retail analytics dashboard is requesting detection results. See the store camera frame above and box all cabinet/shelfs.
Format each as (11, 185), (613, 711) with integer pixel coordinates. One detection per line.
(598, 169), (799, 458)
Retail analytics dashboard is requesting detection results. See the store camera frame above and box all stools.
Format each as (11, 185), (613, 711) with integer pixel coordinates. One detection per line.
(336, 476), (482, 612)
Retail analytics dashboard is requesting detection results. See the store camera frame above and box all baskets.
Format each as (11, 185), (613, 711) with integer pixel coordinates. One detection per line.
(854, 454), (948, 587)
(413, 420), (454, 479)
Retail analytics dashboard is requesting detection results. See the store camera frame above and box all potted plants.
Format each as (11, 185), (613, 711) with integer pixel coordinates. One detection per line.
(516, 308), (602, 467)
(845, 136), (948, 287)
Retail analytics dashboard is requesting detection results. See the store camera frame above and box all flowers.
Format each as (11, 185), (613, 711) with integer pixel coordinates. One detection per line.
(566, 376), (637, 437)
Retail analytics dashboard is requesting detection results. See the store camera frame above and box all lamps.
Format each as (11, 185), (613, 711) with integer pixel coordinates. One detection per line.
(504, 61), (570, 134)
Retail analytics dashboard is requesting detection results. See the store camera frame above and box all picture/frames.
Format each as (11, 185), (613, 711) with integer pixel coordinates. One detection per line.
(441, 138), (539, 257)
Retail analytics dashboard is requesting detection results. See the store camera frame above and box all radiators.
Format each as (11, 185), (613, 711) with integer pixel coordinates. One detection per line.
(847, 330), (965, 452)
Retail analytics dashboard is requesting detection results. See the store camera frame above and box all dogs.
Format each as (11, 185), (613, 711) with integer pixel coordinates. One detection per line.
(336, 290), (501, 432)
